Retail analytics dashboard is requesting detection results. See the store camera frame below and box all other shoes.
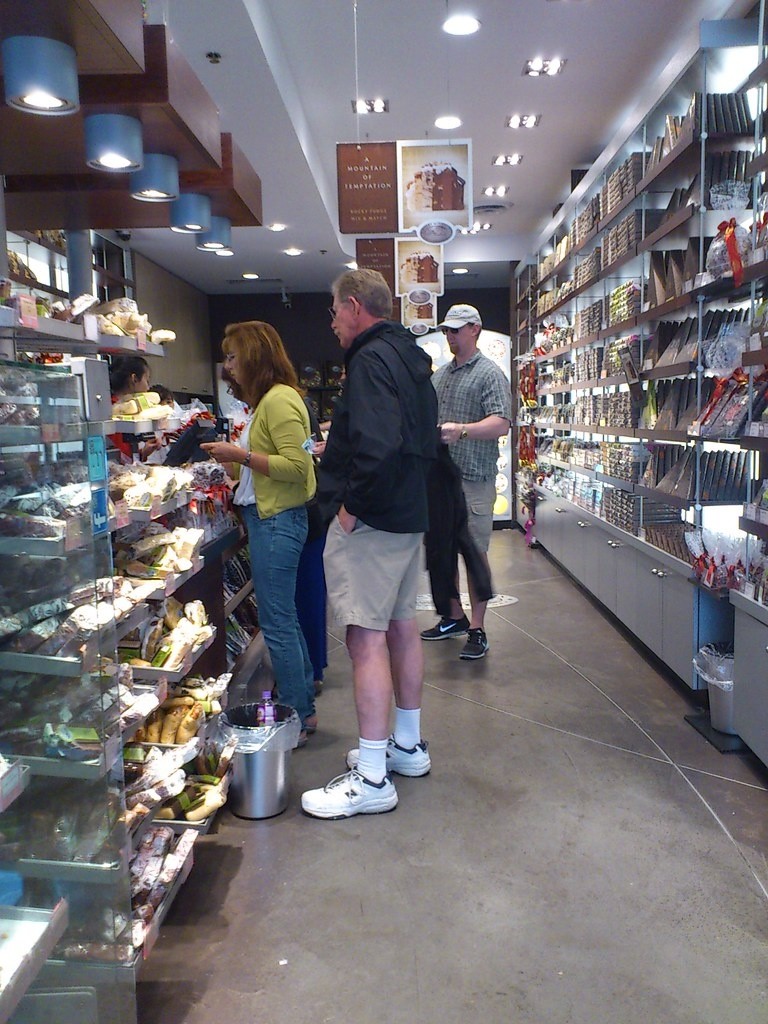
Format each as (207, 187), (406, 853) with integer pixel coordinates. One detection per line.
(293, 711), (317, 749)
(271, 681), (323, 698)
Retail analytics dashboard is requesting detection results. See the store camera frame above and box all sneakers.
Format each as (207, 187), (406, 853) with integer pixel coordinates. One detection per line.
(345, 734), (431, 778)
(459, 628), (489, 659)
(420, 614), (470, 640)
(301, 766), (398, 819)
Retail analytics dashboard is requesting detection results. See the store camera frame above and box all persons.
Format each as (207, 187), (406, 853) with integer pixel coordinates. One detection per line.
(420, 304), (512, 660)
(300, 269), (440, 819)
(200, 320), (346, 750)
(106, 355), (174, 466)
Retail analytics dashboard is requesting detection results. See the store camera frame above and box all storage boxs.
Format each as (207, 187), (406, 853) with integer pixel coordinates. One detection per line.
(536, 92), (768, 566)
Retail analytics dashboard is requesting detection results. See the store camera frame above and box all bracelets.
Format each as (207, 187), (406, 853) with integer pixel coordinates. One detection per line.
(244, 452), (251, 467)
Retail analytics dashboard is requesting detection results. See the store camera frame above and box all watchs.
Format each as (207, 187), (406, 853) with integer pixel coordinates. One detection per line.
(459, 424), (467, 440)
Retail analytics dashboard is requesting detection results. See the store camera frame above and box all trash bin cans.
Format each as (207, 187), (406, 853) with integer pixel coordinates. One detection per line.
(700, 642), (735, 735)
(219, 703), (297, 821)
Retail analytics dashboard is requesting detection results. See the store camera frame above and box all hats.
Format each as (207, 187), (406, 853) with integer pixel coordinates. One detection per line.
(436, 305), (482, 328)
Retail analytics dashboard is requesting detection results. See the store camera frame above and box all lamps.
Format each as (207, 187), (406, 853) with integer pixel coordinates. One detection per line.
(195, 216), (232, 252)
(81, 113), (144, 174)
(128, 152), (181, 203)
(0, 34), (81, 117)
(168, 193), (212, 234)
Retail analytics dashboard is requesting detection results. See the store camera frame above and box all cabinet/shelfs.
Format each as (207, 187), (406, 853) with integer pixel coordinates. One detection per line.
(0, 305), (235, 986)
(726, 0), (768, 769)
(7, 229), (136, 301)
(223, 578), (275, 702)
(513, 14), (768, 695)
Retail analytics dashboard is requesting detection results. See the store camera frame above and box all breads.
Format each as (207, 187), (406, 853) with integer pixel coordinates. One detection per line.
(0, 281), (228, 968)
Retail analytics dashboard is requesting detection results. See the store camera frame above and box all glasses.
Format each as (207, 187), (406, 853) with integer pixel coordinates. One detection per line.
(328, 300), (349, 320)
(226, 385), (233, 395)
(442, 328), (458, 335)
(227, 353), (238, 361)
